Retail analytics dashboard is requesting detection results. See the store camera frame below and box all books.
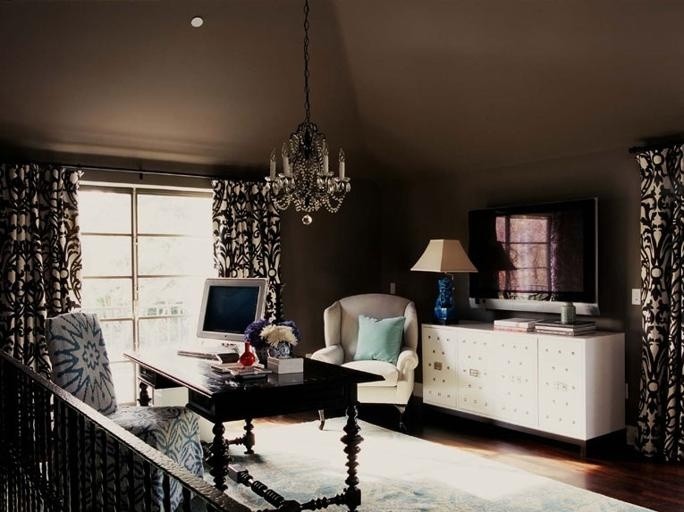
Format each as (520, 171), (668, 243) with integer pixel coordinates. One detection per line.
(494, 317), (542, 332)
(535, 318), (597, 337)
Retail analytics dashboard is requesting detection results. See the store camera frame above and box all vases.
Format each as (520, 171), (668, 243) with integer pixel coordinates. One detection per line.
(256, 347), (268, 364)
(269, 346), (289, 360)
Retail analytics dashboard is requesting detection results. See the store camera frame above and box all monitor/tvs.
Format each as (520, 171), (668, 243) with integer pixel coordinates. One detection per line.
(175, 277), (268, 358)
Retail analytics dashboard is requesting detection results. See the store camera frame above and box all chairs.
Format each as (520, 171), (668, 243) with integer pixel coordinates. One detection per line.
(310, 293), (418, 431)
(43, 312), (204, 512)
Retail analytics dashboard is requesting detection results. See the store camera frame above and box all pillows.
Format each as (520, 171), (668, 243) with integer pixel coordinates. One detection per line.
(354, 312), (406, 366)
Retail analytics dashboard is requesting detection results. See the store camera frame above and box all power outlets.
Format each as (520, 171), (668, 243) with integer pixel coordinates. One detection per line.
(631, 288), (641, 305)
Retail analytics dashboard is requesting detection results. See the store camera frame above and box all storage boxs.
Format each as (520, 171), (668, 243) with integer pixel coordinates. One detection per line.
(422, 323), (626, 459)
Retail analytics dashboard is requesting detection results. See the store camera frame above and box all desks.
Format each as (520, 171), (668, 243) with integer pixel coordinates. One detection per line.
(123, 340), (386, 512)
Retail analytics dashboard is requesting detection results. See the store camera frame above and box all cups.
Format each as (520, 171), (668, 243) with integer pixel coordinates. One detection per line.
(561, 306), (576, 324)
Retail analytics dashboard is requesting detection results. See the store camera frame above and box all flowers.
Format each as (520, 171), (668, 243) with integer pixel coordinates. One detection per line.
(259, 326), (297, 345)
(278, 320), (303, 342)
(244, 320), (270, 346)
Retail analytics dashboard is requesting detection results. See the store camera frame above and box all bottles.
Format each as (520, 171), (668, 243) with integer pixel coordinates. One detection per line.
(561, 302), (576, 324)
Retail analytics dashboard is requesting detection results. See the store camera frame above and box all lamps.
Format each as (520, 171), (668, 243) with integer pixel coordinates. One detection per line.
(265, 0), (351, 225)
(410, 239), (478, 322)
